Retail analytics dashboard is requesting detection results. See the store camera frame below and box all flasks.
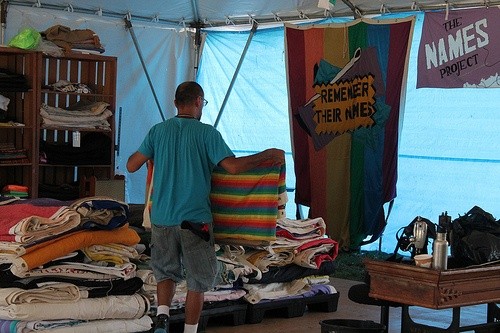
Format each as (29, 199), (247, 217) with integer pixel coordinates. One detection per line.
(432, 226), (449, 271)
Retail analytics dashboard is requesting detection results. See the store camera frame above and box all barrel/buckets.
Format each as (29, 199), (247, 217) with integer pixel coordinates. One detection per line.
(319, 319), (387, 333)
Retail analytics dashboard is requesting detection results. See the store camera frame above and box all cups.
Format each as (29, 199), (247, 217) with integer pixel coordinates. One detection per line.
(414, 254), (433, 268)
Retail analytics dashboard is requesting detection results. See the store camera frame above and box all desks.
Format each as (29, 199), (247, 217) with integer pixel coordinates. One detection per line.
(368, 255), (500, 333)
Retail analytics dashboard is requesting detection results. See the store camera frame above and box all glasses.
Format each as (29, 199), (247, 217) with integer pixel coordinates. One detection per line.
(196, 96), (208, 106)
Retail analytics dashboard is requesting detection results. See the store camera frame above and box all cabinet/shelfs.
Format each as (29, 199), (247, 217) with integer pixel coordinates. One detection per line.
(0, 46), (117, 203)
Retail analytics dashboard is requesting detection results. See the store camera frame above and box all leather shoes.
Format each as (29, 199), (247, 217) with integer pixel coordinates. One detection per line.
(154, 314), (169, 333)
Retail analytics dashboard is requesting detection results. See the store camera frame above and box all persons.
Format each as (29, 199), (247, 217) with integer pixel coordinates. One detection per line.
(126, 81), (285, 333)
